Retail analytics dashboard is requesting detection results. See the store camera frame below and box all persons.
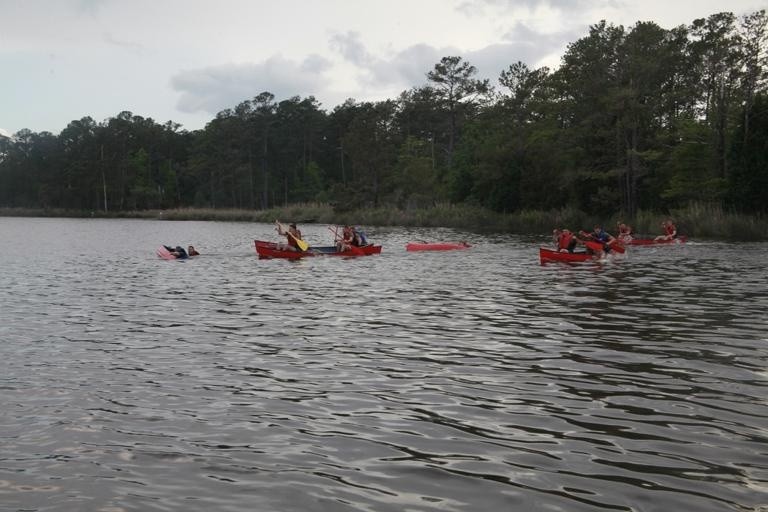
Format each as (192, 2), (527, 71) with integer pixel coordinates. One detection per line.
(273, 220), (302, 253)
(550, 220), (634, 260)
(333, 224), (370, 254)
(652, 218), (676, 241)
(186, 246), (199, 256)
(166, 245), (187, 259)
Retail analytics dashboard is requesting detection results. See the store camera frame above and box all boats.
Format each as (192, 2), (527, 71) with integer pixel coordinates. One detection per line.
(156, 244), (177, 260)
(253, 240), (382, 259)
(539, 235), (687, 266)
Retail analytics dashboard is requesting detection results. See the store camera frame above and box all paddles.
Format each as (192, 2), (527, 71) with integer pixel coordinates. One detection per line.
(663, 223), (675, 244)
(580, 230), (625, 254)
(555, 230), (603, 251)
(327, 226), (365, 256)
(276, 220), (309, 252)
